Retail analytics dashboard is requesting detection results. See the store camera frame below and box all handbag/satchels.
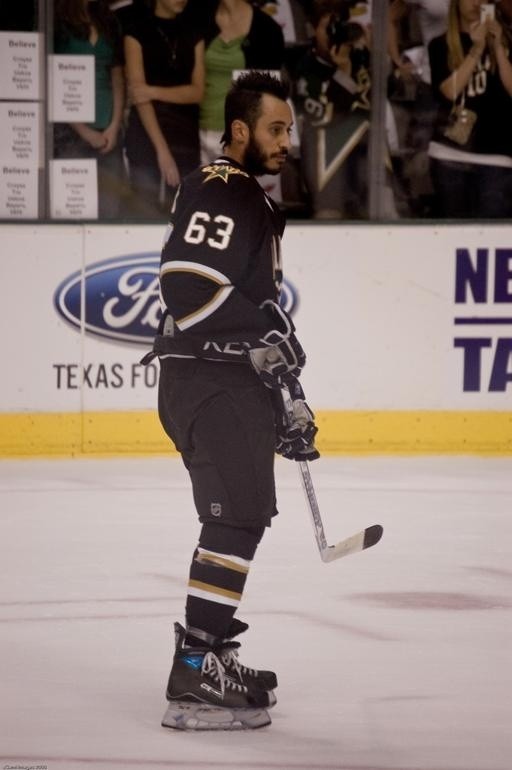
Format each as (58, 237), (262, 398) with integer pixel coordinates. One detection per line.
(443, 105), (477, 144)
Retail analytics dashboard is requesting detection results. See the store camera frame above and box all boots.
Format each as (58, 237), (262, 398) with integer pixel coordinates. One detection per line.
(166, 618), (278, 708)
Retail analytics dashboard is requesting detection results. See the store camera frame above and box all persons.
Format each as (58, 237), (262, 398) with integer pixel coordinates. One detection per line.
(49, 1), (510, 219)
(140, 70), (322, 732)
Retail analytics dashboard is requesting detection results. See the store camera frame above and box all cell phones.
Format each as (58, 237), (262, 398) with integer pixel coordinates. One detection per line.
(481, 3), (495, 26)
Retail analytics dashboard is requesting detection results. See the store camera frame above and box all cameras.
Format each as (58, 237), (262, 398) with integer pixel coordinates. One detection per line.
(327, 10), (362, 51)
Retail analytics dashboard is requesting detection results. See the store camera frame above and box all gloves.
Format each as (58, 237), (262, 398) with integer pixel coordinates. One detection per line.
(247, 299), (320, 462)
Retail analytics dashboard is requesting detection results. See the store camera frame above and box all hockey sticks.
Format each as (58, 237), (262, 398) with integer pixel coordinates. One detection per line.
(265, 343), (382, 563)
